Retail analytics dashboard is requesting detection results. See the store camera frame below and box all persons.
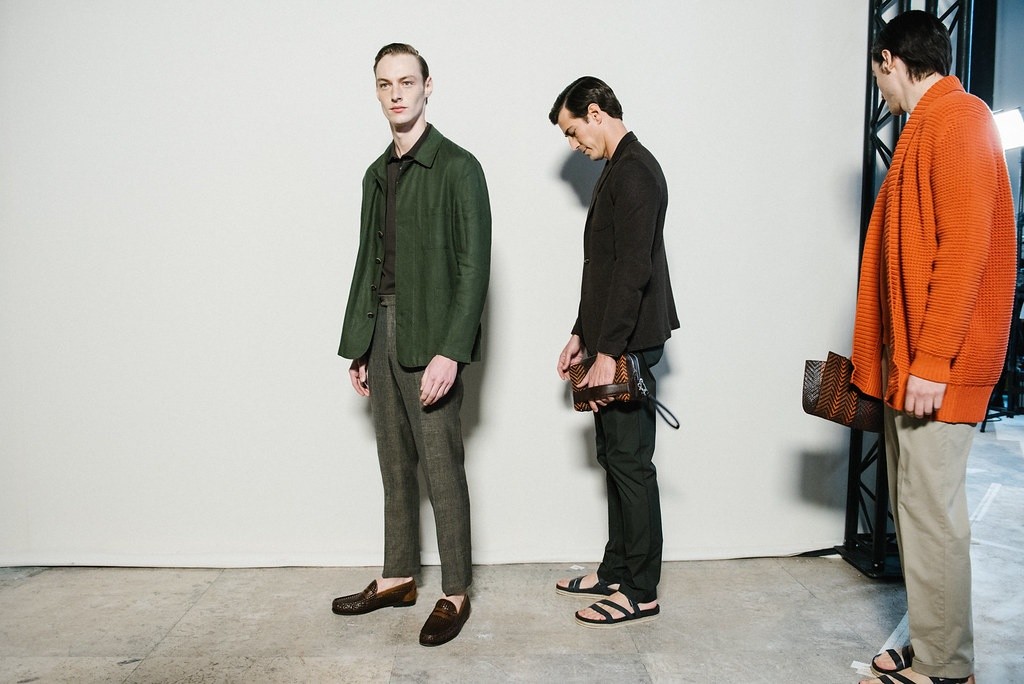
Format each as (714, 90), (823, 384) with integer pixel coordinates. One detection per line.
(845, 9), (1018, 684)
(331, 43), (491, 646)
(547, 75), (680, 630)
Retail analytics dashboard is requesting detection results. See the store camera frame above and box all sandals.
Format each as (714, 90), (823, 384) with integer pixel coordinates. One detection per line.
(871, 645), (915, 676)
(575, 591), (659, 629)
(858, 673), (977, 684)
(555, 573), (617, 596)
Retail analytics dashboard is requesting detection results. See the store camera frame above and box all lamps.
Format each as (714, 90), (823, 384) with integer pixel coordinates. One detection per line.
(992, 106), (1024, 150)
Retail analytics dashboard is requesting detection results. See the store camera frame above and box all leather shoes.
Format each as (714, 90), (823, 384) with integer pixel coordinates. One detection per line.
(333, 578), (416, 614)
(419, 594), (470, 646)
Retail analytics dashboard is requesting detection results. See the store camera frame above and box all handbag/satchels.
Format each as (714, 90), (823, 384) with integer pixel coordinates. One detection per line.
(569, 354), (681, 432)
